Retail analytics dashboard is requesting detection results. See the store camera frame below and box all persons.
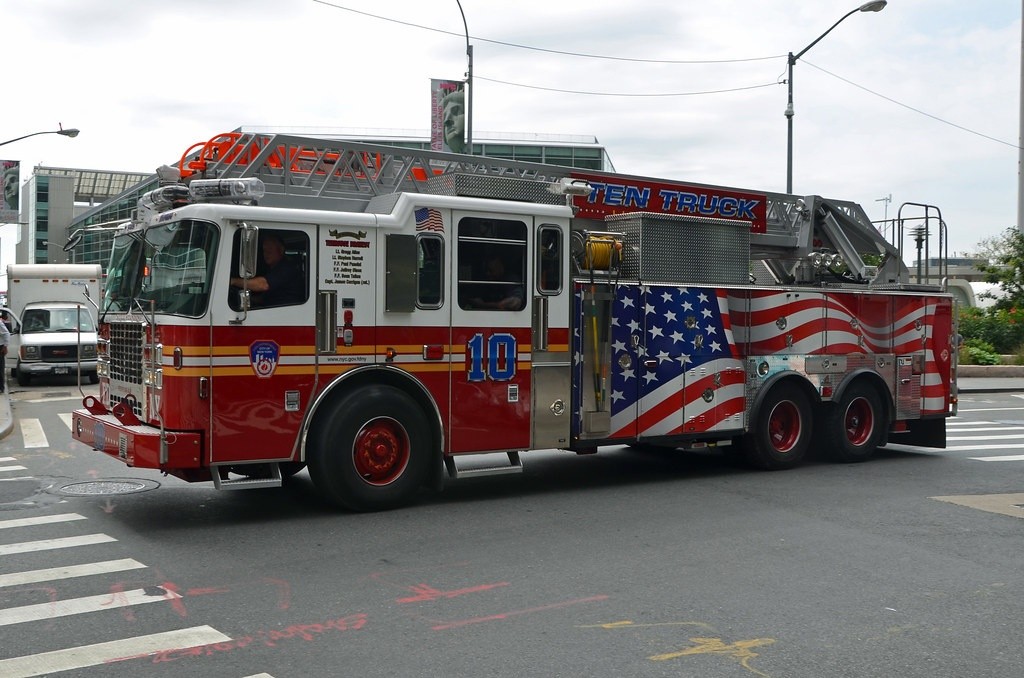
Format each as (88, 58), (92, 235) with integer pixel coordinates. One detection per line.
(229, 238), (299, 311)
(469, 259), (521, 313)
(0, 318), (11, 394)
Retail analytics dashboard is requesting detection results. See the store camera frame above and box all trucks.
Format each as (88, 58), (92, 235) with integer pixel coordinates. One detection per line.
(0, 262), (106, 385)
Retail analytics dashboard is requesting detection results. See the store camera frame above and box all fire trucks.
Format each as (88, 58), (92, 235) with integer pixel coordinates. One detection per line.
(67, 125), (966, 512)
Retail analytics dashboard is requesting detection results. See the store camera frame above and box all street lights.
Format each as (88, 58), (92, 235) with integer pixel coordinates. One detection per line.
(786, 0), (890, 194)
